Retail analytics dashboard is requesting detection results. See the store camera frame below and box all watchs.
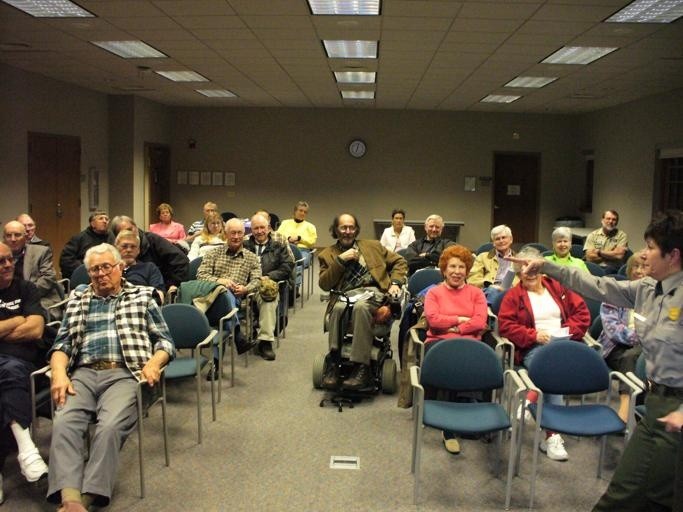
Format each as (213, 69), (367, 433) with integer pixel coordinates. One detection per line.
(390, 280), (399, 285)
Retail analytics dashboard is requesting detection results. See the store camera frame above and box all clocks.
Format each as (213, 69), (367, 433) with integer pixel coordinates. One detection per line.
(348, 140), (367, 158)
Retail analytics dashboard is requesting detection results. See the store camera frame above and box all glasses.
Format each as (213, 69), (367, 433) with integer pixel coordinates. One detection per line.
(87, 262), (121, 274)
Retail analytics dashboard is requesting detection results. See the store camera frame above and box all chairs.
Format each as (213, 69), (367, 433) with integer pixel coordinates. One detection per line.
(522, 243), (548, 254)
(501, 332), (593, 442)
(397, 249), (408, 258)
(177, 283), (239, 402)
(476, 243), (494, 258)
(514, 340), (643, 509)
(30, 363), (51, 445)
(58, 279), (70, 297)
(289, 244), (307, 314)
(160, 304), (218, 445)
(586, 314), (619, 405)
(277, 280), (289, 348)
(410, 327), (504, 421)
(70, 264), (91, 290)
(626, 352), (646, 424)
(584, 262), (604, 277)
(168, 290), (176, 304)
(409, 338), (527, 511)
(405, 269), (444, 307)
(46, 298), (69, 326)
(602, 274), (627, 280)
(46, 364), (170, 498)
(189, 257), (256, 368)
(275, 222), (317, 300)
(487, 289), (510, 334)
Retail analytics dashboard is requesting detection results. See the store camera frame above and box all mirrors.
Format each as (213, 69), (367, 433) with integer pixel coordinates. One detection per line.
(464, 176), (476, 192)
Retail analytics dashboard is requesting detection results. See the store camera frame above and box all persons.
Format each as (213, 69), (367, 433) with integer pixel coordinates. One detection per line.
(243, 216), (295, 361)
(59, 211), (118, 294)
(2, 220), (65, 336)
(583, 208), (628, 274)
(499, 245), (592, 461)
(318, 214), (407, 386)
(150, 202), (186, 242)
(196, 217), (262, 381)
(277, 200), (317, 299)
(188, 213), (227, 260)
(111, 216), (190, 287)
(540, 226), (592, 274)
(187, 201), (224, 237)
(503, 211), (683, 512)
(404, 213), (455, 281)
(425, 244), (487, 454)
(42, 243), (175, 511)
(1, 242), (50, 504)
(114, 230), (164, 291)
(16, 214), (51, 247)
(466, 224), (519, 333)
(246, 210), (296, 266)
(598, 251), (657, 434)
(379, 209), (416, 254)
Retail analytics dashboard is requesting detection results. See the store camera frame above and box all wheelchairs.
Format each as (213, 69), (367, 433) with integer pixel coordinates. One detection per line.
(313, 291), (403, 412)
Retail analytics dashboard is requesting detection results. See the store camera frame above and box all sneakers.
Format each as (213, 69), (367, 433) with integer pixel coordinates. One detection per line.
(540, 433), (569, 461)
(17, 448), (49, 482)
(517, 399), (531, 424)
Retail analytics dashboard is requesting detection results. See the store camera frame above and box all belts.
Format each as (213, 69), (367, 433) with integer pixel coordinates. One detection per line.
(80, 361), (127, 370)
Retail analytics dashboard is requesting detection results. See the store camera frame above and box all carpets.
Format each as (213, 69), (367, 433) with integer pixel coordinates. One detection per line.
(0, 247), (619, 511)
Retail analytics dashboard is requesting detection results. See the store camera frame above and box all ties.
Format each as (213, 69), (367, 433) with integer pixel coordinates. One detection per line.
(258, 244), (262, 256)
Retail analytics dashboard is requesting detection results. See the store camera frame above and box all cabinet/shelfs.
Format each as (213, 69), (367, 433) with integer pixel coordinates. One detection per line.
(373, 221), (465, 242)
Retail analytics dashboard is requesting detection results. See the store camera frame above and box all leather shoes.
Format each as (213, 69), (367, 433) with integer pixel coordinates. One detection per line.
(259, 342), (275, 360)
(56, 502), (88, 512)
(442, 431), (460, 454)
(323, 369), (336, 387)
(343, 368), (369, 389)
(237, 341), (257, 355)
(207, 368), (218, 380)
